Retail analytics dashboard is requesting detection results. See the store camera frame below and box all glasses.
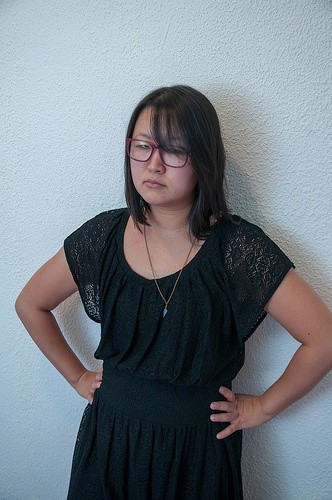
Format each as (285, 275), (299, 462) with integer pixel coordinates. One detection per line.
(126, 136), (193, 168)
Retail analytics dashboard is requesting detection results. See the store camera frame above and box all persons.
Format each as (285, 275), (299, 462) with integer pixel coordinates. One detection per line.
(15, 84), (332, 500)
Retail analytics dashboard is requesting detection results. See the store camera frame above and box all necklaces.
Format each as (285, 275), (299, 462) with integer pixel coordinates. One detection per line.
(143, 226), (196, 316)
